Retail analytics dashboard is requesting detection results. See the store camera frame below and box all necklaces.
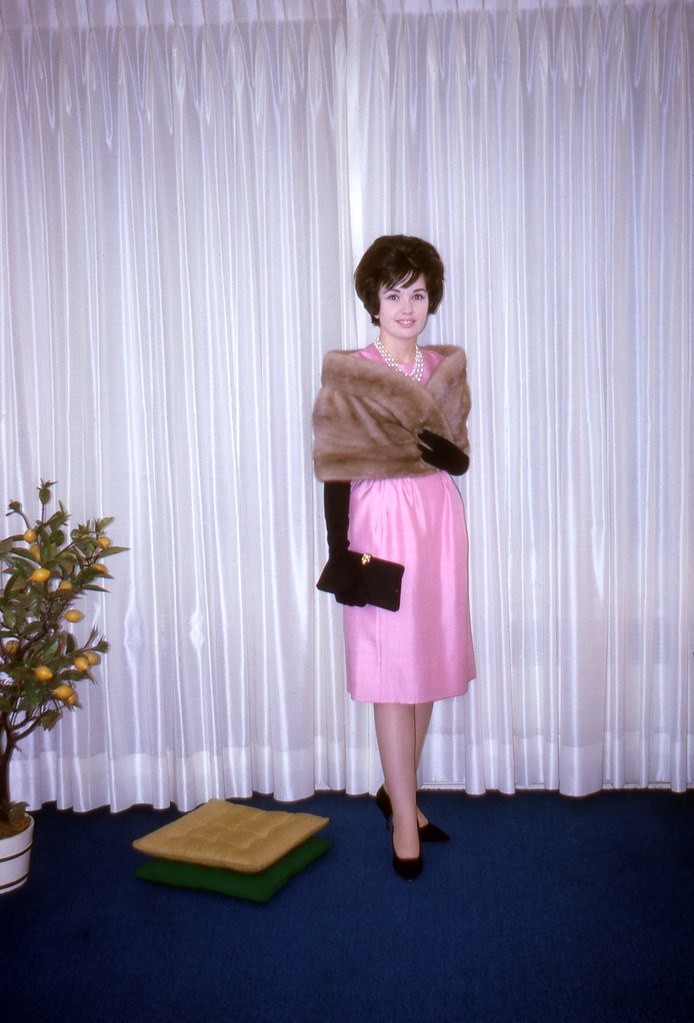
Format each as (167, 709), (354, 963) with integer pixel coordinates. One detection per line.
(375, 334), (425, 383)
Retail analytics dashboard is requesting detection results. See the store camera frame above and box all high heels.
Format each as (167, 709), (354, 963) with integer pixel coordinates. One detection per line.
(391, 824), (423, 882)
(375, 787), (452, 844)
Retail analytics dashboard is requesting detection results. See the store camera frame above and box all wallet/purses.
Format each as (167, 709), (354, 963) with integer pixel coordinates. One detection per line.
(317, 548), (405, 612)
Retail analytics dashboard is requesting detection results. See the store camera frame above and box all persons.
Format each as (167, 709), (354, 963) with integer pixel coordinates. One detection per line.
(312, 234), (476, 881)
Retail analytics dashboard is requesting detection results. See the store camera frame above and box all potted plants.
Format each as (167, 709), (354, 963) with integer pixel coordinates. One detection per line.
(0, 478), (131, 895)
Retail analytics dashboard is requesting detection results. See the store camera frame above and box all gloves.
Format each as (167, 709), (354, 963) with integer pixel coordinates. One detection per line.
(416, 429), (468, 475)
(329, 550), (366, 607)
